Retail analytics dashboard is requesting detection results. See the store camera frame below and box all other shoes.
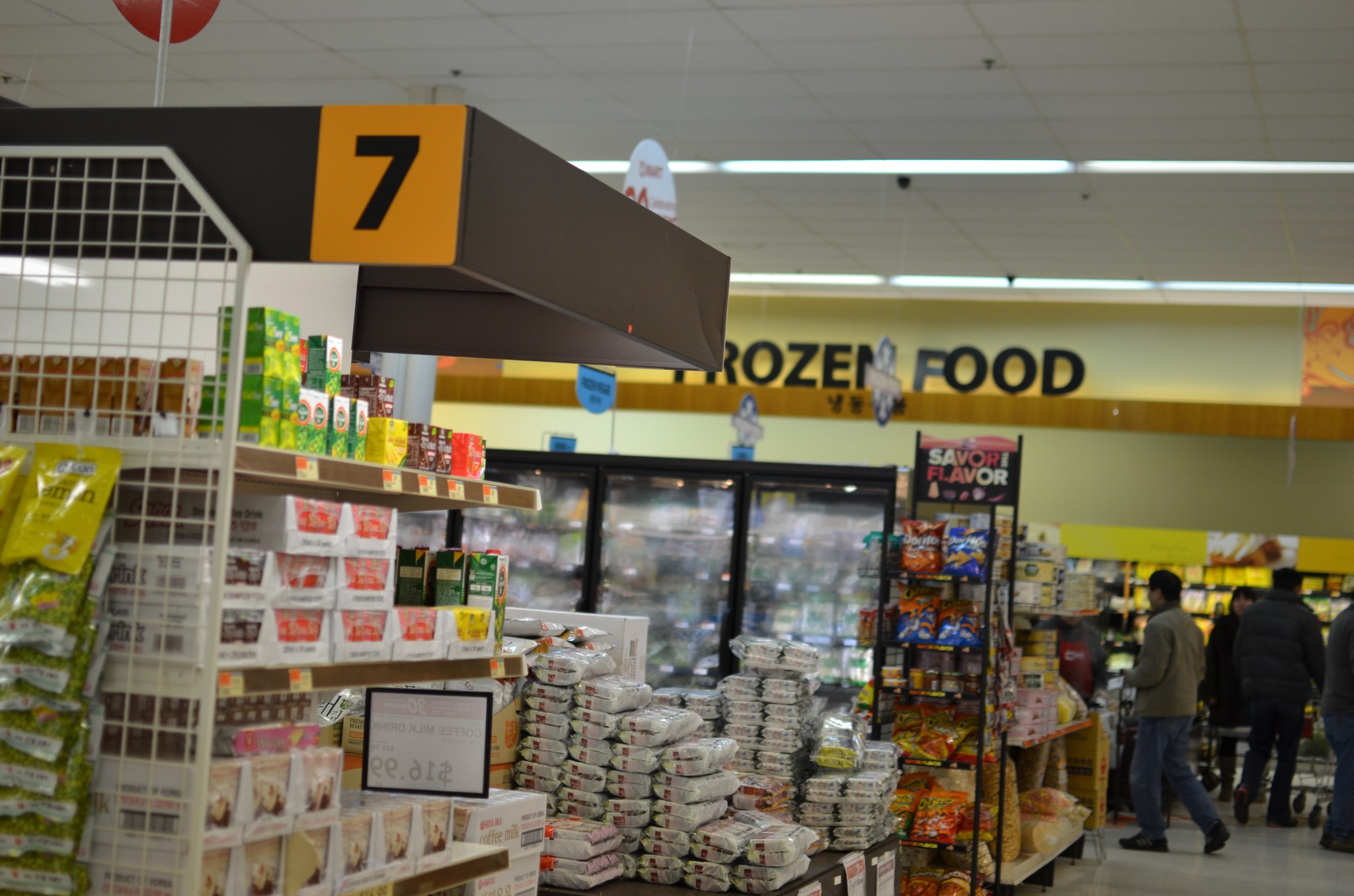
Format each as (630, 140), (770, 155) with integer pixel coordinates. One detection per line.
(1320, 830), (1354, 854)
(1119, 833), (1167, 853)
(1218, 786), (1233, 802)
(1233, 784), (1252, 824)
(1267, 812), (1298, 828)
(1204, 827), (1230, 854)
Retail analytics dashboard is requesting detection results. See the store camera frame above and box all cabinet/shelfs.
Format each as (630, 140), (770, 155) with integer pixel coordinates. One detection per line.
(3, 434), (544, 896)
(857, 429), (1092, 896)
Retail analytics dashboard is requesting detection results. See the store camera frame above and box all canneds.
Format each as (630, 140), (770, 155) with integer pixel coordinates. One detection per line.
(908, 668), (980, 694)
(1008, 628), (1058, 741)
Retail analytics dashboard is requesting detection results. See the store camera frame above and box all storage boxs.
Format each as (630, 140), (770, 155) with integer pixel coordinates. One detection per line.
(105, 499), (496, 657)
(0, 307), (487, 480)
(454, 789), (544, 859)
(504, 607), (650, 681)
(437, 852), (540, 896)
(1015, 555), (1108, 828)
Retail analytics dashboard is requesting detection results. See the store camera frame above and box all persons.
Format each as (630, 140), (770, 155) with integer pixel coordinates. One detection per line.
(1198, 585), (1268, 804)
(1320, 605), (1354, 854)
(1116, 569), (1231, 855)
(1230, 566), (1326, 827)
